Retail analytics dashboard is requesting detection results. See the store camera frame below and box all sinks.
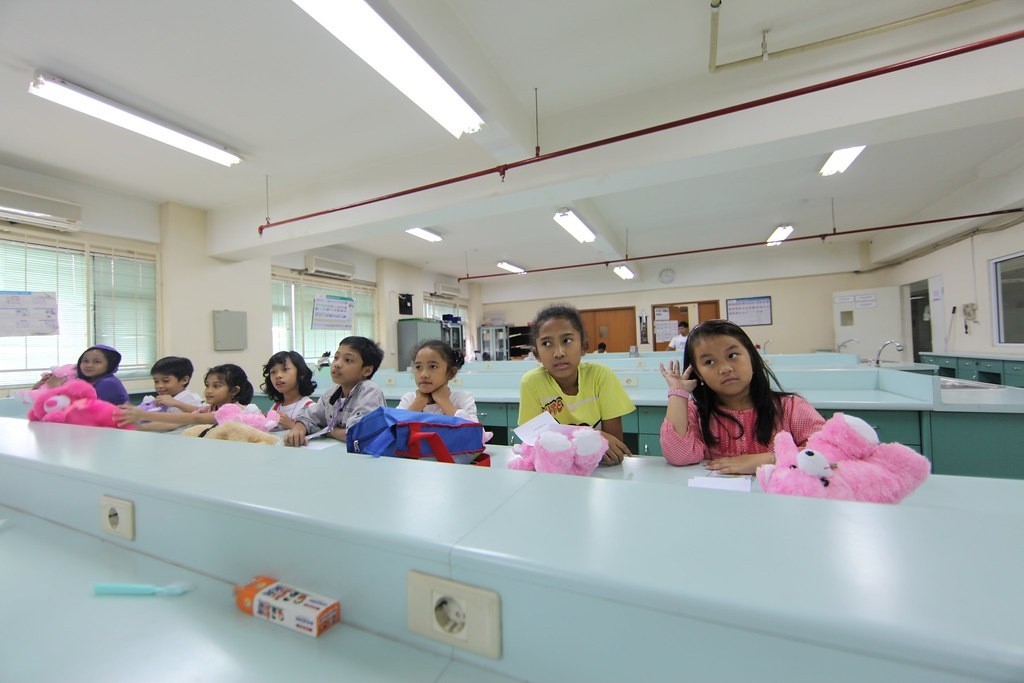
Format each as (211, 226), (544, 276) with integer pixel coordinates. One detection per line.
(940, 381), (1006, 391)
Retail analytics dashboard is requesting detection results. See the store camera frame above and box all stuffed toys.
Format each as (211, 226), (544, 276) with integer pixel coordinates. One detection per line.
(183, 403), (282, 448)
(755, 412), (933, 504)
(26, 363), (136, 432)
(508, 424), (609, 477)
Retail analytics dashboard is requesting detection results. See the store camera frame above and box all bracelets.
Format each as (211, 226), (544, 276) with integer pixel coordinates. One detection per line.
(667, 389), (690, 399)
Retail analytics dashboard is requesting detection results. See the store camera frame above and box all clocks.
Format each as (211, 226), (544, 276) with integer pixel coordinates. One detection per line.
(659, 269), (676, 284)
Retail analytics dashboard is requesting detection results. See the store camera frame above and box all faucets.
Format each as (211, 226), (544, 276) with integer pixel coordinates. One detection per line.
(837, 338), (857, 353)
(763, 339), (773, 355)
(874, 340), (904, 367)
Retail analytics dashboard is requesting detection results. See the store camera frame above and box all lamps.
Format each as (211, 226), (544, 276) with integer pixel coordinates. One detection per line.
(766, 146), (868, 245)
(290, 1), (486, 138)
(407, 208), (635, 280)
(29, 72), (241, 167)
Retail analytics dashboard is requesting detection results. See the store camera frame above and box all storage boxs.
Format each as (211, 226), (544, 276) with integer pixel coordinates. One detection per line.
(236, 576), (340, 637)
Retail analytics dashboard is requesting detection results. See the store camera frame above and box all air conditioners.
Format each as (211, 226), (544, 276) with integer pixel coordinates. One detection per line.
(434, 283), (461, 298)
(305, 256), (355, 280)
(0, 190), (80, 231)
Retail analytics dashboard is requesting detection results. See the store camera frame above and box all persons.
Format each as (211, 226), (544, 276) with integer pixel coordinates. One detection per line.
(32, 344), (131, 406)
(114, 356), (254, 432)
(516, 305), (637, 465)
(395, 340), (477, 419)
(593, 343), (607, 353)
(661, 318), (825, 477)
(259, 351), (317, 430)
(283, 335), (387, 448)
(665, 322), (690, 351)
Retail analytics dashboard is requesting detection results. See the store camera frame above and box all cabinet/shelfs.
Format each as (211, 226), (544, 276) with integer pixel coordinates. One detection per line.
(481, 327), (508, 361)
(919, 352), (1024, 389)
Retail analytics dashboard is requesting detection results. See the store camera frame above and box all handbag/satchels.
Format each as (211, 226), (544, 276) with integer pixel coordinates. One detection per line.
(346, 406), (491, 467)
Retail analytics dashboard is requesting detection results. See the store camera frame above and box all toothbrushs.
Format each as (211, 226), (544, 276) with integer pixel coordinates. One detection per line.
(94, 580), (192, 597)
